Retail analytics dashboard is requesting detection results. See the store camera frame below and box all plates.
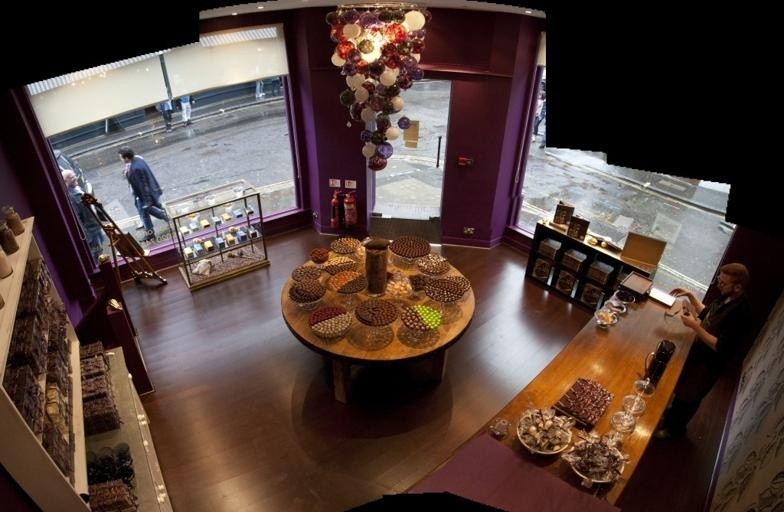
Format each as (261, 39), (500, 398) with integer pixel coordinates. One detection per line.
(516, 412), (573, 455)
(569, 438), (626, 483)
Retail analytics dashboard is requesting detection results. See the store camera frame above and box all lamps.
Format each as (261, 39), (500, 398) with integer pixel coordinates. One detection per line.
(325, 1), (433, 171)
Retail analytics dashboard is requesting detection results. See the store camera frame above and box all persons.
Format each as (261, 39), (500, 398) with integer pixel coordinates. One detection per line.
(123, 154), (166, 230)
(533, 81), (547, 137)
(117, 146), (170, 242)
(155, 100), (176, 133)
(61, 169), (105, 265)
(272, 77), (280, 96)
(655, 263), (753, 442)
(176, 96), (196, 127)
(256, 80), (266, 98)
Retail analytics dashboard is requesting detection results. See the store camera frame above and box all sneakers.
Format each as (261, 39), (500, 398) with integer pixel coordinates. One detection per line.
(655, 430), (685, 445)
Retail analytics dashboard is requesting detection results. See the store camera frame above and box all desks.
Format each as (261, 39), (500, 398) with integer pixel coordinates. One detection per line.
(404, 287), (702, 512)
(280, 240), (476, 403)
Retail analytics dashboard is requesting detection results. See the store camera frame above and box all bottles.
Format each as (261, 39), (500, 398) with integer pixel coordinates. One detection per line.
(638, 341), (676, 395)
(0, 205), (25, 310)
(364, 247), (388, 297)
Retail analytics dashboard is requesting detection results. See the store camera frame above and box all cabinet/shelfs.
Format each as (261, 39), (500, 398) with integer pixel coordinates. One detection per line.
(0, 215), (172, 512)
(162, 179), (270, 293)
(524, 216), (658, 316)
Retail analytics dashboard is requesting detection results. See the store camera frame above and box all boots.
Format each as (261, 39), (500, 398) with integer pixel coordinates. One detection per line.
(139, 229), (155, 242)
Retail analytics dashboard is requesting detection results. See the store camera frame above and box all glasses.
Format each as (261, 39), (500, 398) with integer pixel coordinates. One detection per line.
(717, 277), (736, 287)
